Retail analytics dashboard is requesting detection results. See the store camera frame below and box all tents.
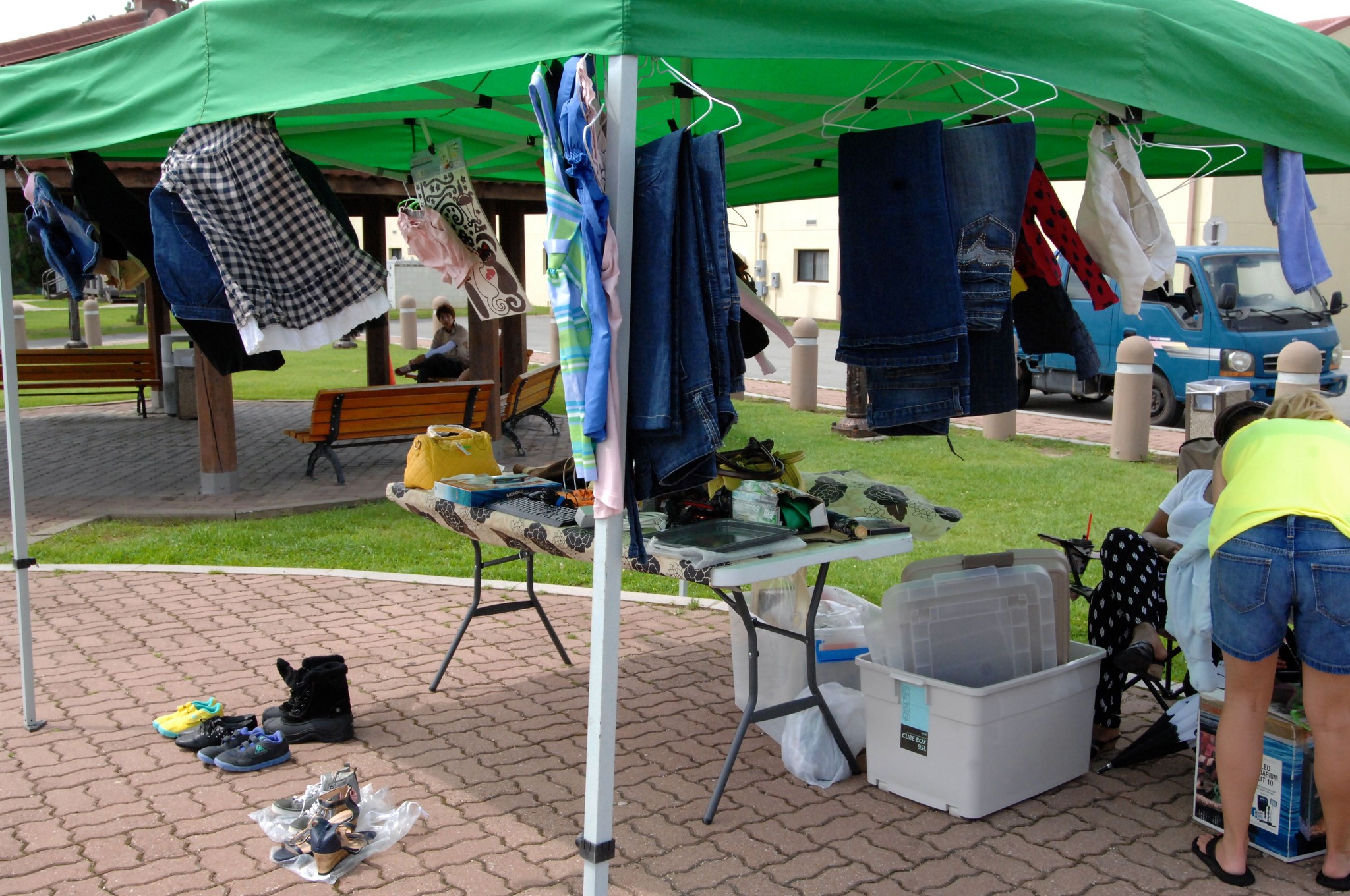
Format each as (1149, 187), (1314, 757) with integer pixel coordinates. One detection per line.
(0, 0), (1350, 896)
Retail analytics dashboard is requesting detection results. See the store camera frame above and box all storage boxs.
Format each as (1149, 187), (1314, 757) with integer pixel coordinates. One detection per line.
(727, 584), (881, 747)
(854, 640), (1107, 820)
(1192, 692), (1327, 862)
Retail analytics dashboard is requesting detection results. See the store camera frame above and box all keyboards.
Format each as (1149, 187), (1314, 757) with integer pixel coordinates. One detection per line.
(486, 497), (578, 527)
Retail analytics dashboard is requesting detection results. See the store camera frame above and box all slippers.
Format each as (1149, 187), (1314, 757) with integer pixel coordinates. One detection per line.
(1192, 833), (1255, 886)
(1315, 869), (1350, 892)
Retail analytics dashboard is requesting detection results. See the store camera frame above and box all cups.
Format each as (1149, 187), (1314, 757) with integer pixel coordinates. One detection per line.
(1067, 538), (1095, 575)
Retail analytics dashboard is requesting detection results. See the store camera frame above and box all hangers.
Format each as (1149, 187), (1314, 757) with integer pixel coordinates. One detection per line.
(397, 180), (440, 230)
(583, 54), (742, 192)
(821, 60), (1249, 211)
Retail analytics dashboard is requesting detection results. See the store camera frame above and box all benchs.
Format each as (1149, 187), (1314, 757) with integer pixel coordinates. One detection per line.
(500, 360), (560, 455)
(0, 349), (163, 418)
(283, 380), (494, 483)
(405, 349), (533, 382)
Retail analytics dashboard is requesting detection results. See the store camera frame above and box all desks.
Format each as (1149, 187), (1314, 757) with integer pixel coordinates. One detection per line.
(386, 473), (913, 824)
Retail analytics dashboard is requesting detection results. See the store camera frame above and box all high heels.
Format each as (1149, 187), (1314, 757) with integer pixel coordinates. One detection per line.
(308, 817), (376, 876)
(274, 783), (359, 862)
(1090, 733), (1121, 757)
(1122, 641), (1165, 681)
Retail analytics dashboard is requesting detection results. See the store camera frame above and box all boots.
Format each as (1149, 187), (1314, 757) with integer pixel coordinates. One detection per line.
(261, 653), (344, 723)
(263, 662), (354, 743)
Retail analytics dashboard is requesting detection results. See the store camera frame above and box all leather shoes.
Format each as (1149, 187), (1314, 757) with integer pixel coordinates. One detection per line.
(178, 713), (258, 737)
(175, 720), (255, 751)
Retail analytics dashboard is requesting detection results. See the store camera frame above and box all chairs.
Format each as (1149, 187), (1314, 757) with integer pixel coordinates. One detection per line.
(1185, 270), (1218, 317)
(1036, 429), (1220, 712)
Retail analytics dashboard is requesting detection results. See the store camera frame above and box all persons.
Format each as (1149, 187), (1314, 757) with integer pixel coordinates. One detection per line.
(1088, 400), (1268, 756)
(394, 304), (502, 382)
(1192, 389), (1350, 891)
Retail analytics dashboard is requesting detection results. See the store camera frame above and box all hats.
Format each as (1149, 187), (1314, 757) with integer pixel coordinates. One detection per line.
(1212, 400), (1274, 448)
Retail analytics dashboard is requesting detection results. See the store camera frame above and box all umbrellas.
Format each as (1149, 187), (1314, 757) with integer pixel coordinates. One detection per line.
(1097, 661), (1225, 774)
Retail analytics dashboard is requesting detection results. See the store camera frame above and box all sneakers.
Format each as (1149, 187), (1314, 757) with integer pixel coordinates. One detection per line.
(214, 730), (291, 772)
(198, 727), (265, 765)
(157, 702), (224, 739)
(394, 364), (412, 376)
(272, 763), (351, 816)
(288, 767), (362, 834)
(152, 697), (217, 729)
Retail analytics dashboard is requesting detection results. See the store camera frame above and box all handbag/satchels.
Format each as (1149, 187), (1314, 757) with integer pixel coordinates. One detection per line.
(706, 436), (830, 533)
(404, 424), (502, 491)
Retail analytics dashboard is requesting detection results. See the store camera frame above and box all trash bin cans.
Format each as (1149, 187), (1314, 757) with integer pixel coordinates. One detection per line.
(174, 348), (197, 419)
(161, 334), (194, 418)
(1184, 379), (1251, 439)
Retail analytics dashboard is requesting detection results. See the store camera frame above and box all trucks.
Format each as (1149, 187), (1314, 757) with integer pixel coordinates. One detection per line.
(1014, 245), (1348, 426)
